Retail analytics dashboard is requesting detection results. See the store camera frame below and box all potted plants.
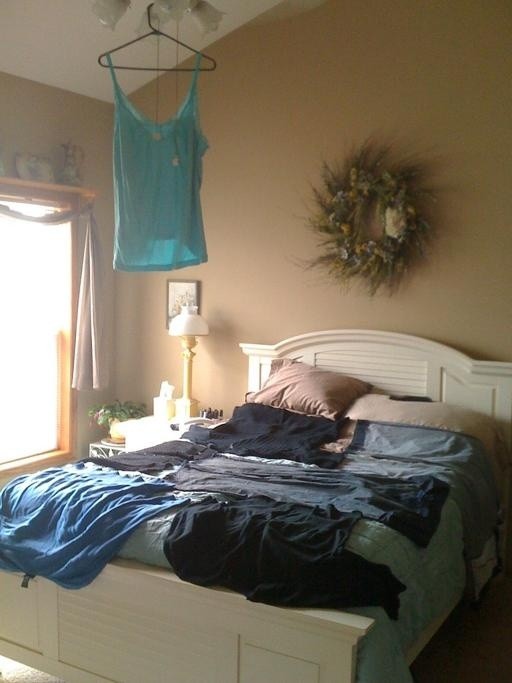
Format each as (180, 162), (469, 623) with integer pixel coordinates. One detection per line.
(87, 399), (147, 444)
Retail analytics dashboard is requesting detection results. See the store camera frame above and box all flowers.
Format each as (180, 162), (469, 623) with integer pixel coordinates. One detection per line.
(298, 131), (449, 298)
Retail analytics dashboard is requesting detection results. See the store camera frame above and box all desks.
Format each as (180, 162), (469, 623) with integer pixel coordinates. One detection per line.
(88, 442), (130, 460)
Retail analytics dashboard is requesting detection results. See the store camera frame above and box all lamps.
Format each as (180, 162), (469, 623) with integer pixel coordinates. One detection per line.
(167, 306), (211, 420)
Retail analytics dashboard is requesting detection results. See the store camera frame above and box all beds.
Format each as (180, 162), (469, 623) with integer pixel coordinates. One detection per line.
(0, 329), (512, 683)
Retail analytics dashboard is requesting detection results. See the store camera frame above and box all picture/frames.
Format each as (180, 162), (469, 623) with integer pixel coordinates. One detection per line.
(165, 279), (200, 330)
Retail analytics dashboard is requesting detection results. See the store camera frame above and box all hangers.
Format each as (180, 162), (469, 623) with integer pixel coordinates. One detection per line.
(95, 2), (218, 72)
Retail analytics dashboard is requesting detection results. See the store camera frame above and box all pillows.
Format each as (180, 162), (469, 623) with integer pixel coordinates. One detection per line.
(244, 357), (504, 451)
